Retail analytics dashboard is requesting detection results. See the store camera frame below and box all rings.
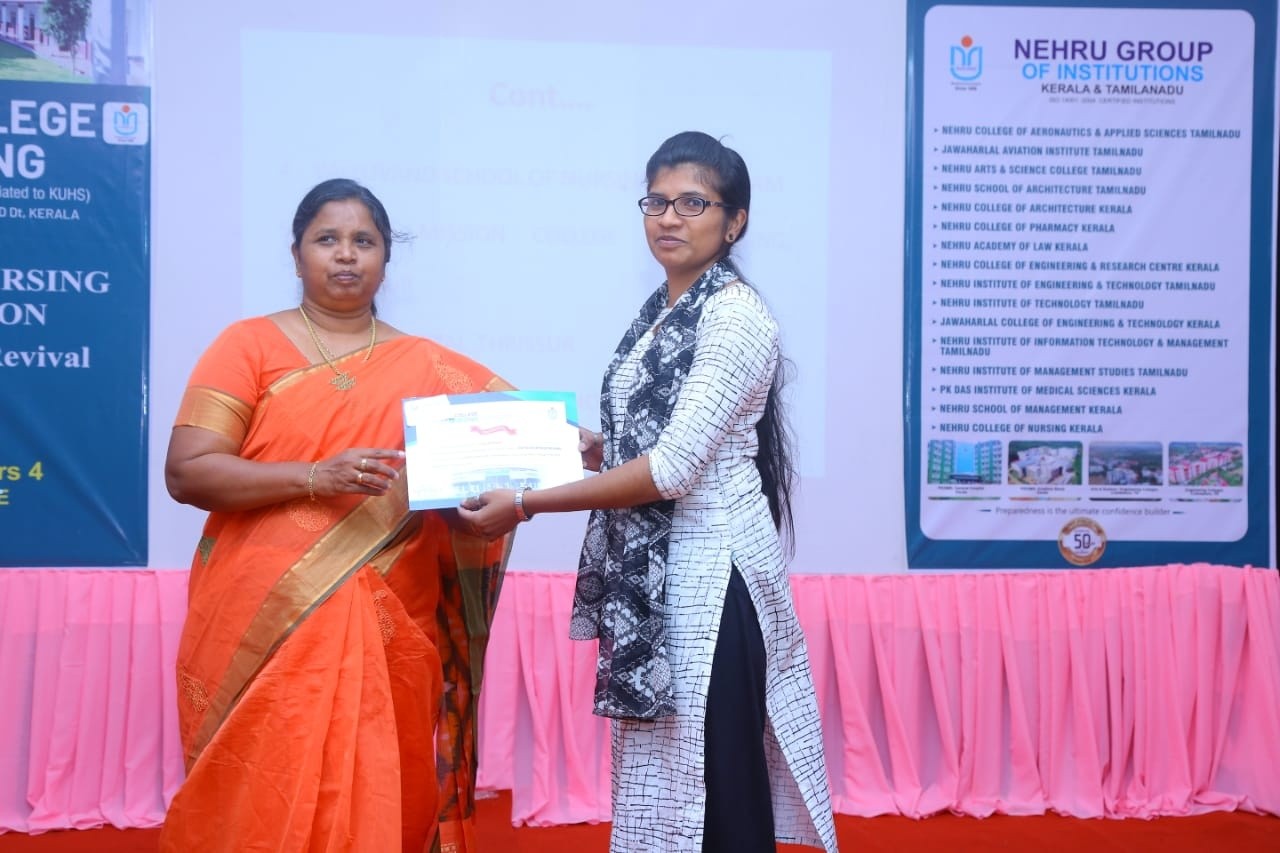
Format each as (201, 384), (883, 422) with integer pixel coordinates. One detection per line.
(357, 473), (363, 483)
(476, 494), (482, 507)
(360, 458), (367, 471)
(592, 433), (599, 447)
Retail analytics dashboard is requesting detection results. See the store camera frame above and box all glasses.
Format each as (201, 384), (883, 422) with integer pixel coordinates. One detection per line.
(638, 197), (733, 217)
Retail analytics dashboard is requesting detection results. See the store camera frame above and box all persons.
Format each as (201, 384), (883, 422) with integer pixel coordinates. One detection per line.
(454, 131), (838, 853)
(159, 176), (523, 853)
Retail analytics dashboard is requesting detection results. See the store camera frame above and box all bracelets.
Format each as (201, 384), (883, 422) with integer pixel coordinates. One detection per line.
(307, 460), (321, 501)
(514, 485), (534, 522)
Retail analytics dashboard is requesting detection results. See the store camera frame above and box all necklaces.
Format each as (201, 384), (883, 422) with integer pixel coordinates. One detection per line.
(298, 304), (376, 391)
